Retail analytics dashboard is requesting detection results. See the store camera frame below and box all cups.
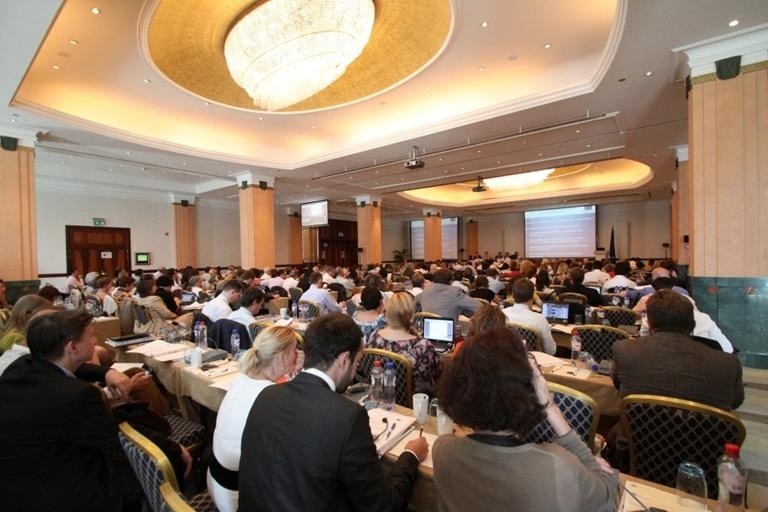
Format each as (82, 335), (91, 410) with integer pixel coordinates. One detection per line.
(191, 348), (202, 369)
(280, 308), (287, 320)
(676, 462), (708, 509)
(412, 393), (429, 425)
(436, 405), (454, 438)
(578, 352), (590, 369)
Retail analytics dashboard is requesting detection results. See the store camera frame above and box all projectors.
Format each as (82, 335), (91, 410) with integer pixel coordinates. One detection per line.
(403, 146), (424, 170)
(471, 178), (489, 192)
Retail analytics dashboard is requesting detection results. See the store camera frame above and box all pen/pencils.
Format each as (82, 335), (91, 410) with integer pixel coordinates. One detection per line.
(418, 425), (425, 437)
(551, 365), (563, 372)
(205, 367), (230, 377)
(385, 422), (397, 438)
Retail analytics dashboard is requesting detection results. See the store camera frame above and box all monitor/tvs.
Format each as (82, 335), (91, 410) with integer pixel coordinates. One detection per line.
(299, 199), (329, 229)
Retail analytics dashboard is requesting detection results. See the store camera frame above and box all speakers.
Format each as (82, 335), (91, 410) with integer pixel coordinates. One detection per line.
(458, 246), (464, 258)
(663, 242), (669, 258)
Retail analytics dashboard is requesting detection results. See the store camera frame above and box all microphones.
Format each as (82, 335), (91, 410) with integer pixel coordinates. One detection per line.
(169, 318), (227, 366)
(139, 304), (187, 342)
(368, 417), (389, 448)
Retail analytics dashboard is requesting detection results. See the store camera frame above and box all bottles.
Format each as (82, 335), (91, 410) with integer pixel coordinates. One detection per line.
(291, 299), (297, 319)
(571, 331), (581, 362)
(370, 362), (383, 404)
(585, 304), (593, 325)
(716, 442), (743, 510)
(615, 285), (623, 295)
(384, 364), (397, 411)
(199, 320), (207, 347)
(194, 324), (202, 348)
(231, 328), (242, 358)
(597, 305), (607, 327)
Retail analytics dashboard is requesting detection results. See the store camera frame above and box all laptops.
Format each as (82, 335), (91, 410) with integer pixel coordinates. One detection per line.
(181, 292), (195, 307)
(542, 301), (569, 326)
(423, 316), (456, 352)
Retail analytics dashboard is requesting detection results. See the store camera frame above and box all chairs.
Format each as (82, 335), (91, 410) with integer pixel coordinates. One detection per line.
(288, 286), (302, 305)
(507, 323), (545, 356)
(86, 294), (102, 312)
(249, 322), (268, 340)
(571, 324), (632, 363)
(158, 481), (196, 512)
(292, 299), (323, 320)
(355, 347), (413, 413)
(624, 392), (748, 501)
(556, 292), (589, 323)
(527, 380), (598, 456)
(589, 304), (637, 328)
(409, 312), (444, 339)
(91, 382), (210, 459)
(601, 292), (627, 307)
(549, 284), (566, 292)
(116, 420), (220, 512)
(116, 296), (137, 337)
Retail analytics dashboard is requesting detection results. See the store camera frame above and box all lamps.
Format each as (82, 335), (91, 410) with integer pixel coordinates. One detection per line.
(221, 0), (376, 113)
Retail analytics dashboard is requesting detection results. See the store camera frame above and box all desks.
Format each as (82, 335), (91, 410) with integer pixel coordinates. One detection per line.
(103, 335), (156, 365)
(252, 314), (626, 418)
(128, 330), (754, 512)
(90, 316), (121, 344)
(453, 320), (576, 349)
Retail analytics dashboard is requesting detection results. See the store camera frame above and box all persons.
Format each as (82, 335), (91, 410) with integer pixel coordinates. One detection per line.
(348, 286), (388, 375)
(110, 267), (127, 285)
(610, 290), (746, 498)
(133, 268), (142, 281)
(499, 279), (559, 357)
(60, 267), (84, 296)
(368, 292), (442, 406)
(162, 269), (183, 293)
(298, 272), (341, 320)
(202, 251), (610, 285)
(431, 328), (620, 512)
(633, 278), (698, 311)
(0, 281), (13, 325)
(198, 281), (241, 324)
(0, 312), (191, 512)
(207, 326), (306, 511)
(557, 268), (602, 307)
(611, 256), (684, 286)
(134, 280), (176, 336)
(0, 294), (171, 420)
(180, 265), (194, 288)
(447, 303), (507, 361)
(414, 269), (486, 329)
(112, 278), (134, 308)
(639, 291), (738, 351)
(154, 275), (182, 313)
(184, 269), (200, 289)
(227, 288), (262, 346)
(236, 311), (429, 511)
(189, 276), (209, 301)
(154, 266), (165, 278)
(90, 277), (118, 317)
(82, 272), (97, 295)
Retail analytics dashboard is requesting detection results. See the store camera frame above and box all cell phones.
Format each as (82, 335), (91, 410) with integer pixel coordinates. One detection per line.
(144, 368), (153, 375)
